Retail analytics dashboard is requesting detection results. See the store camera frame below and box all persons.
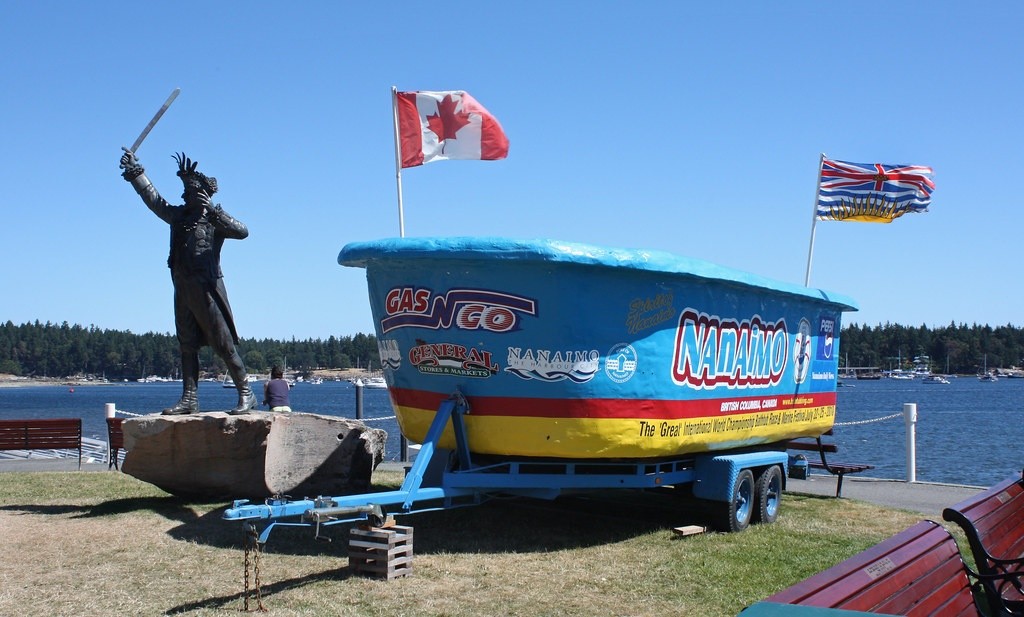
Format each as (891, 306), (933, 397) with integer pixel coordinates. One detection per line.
(120, 146), (258, 414)
(262, 367), (291, 412)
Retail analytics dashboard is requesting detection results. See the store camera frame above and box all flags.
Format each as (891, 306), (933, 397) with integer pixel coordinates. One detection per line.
(816, 157), (935, 224)
(397, 90), (509, 169)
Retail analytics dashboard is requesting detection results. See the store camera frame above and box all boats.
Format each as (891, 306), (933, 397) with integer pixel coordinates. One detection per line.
(336, 234), (858, 461)
(857, 374), (881, 380)
(222, 369), (236, 388)
(346, 378), (387, 390)
(976, 354), (998, 383)
(922, 375), (950, 384)
(297, 375), (324, 385)
(267, 356), (296, 385)
(995, 368), (1024, 378)
(891, 374), (915, 381)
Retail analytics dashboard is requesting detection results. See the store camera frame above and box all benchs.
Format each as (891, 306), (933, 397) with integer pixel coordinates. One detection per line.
(942, 471), (1024, 612)
(105, 417), (125, 472)
(740, 519), (1024, 617)
(786, 441), (875, 500)
(0, 417), (83, 472)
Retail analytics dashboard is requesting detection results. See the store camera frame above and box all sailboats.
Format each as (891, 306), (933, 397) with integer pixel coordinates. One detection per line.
(910, 354), (933, 378)
